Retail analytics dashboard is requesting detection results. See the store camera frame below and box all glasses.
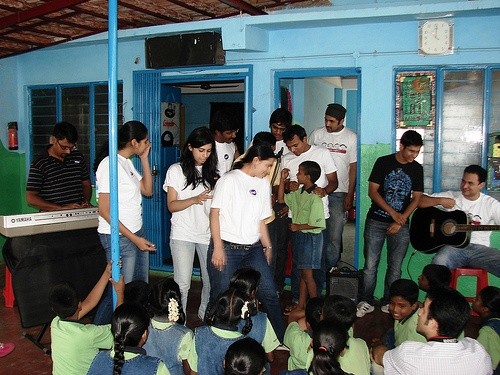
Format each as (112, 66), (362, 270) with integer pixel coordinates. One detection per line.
(57, 140), (78, 150)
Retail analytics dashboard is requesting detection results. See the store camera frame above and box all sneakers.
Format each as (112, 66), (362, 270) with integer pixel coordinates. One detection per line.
(356, 300), (374, 318)
(0, 342), (15, 357)
(283, 302), (298, 315)
(381, 304), (391, 314)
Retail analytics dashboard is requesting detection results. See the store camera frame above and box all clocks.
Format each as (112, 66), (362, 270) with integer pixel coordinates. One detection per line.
(416, 17), (455, 56)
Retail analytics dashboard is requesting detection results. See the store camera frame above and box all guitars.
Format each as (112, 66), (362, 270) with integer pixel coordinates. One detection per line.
(409, 206), (500, 254)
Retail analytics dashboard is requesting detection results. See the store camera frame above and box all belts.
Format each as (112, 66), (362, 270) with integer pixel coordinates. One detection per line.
(224, 241), (251, 250)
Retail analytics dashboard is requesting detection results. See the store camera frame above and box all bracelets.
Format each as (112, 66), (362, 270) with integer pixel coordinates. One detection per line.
(262, 247), (273, 249)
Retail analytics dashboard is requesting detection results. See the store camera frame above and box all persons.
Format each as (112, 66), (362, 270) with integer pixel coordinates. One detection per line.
(355, 130), (424, 317)
(51, 255), (125, 375)
(209, 109), (240, 184)
(280, 123), (339, 295)
(187, 268), (281, 375)
(93, 121), (158, 325)
(278, 160), (326, 316)
(162, 125), (220, 327)
(85, 277), (194, 374)
(371, 262), (500, 375)
(415, 164), (500, 278)
(25, 120), (93, 282)
(205, 131), (289, 350)
(234, 107), (291, 163)
(306, 103), (358, 269)
(282, 294), (371, 375)
(0, 342), (15, 358)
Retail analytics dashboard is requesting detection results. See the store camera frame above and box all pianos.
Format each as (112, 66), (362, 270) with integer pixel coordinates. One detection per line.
(0, 207), (98, 237)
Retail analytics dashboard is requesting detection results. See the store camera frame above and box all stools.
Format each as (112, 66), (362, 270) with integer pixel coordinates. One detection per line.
(448, 266), (489, 317)
